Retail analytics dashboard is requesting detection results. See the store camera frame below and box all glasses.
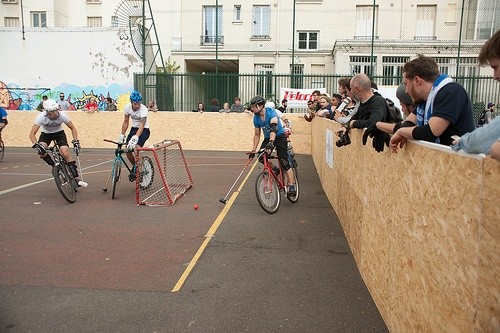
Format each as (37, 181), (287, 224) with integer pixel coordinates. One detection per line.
(60, 96), (64, 98)
(307, 104), (311, 105)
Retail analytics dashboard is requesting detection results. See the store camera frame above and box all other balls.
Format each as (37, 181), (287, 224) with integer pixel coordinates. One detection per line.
(194, 205), (197, 209)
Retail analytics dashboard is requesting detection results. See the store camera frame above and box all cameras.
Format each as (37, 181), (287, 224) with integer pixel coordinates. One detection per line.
(336, 131), (351, 147)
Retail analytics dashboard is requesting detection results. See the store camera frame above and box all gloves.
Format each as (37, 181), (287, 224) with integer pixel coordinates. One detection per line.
(72, 139), (82, 154)
(362, 122), (381, 145)
(32, 142), (47, 155)
(127, 135), (138, 151)
(118, 133), (125, 144)
(373, 131), (390, 153)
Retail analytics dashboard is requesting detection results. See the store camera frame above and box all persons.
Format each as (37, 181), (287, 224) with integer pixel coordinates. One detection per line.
(0, 107), (8, 132)
(29, 99), (81, 178)
(451, 29), (500, 160)
(389, 55), (475, 154)
(219, 103), (230, 113)
(304, 52), (417, 155)
(147, 101), (158, 112)
(36, 92), (119, 111)
(191, 103), (206, 113)
(119, 90), (150, 183)
(244, 97), (290, 114)
(481, 102), (495, 124)
(249, 95), (298, 198)
(207, 98), (220, 112)
(230, 97), (245, 113)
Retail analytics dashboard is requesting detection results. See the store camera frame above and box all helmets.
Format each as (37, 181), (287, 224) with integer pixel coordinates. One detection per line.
(250, 96), (266, 105)
(130, 90), (143, 103)
(43, 99), (59, 111)
(396, 83), (415, 105)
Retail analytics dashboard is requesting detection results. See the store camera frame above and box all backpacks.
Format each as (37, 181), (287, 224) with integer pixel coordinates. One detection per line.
(479, 110), (489, 125)
(384, 98), (402, 122)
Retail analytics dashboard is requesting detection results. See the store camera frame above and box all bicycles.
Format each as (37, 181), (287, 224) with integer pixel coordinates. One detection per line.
(245, 138), (299, 214)
(32, 141), (81, 203)
(0, 132), (5, 162)
(103, 139), (155, 199)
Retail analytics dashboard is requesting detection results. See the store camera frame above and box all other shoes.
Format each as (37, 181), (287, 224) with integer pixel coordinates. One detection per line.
(141, 161), (149, 175)
(75, 178), (82, 184)
(128, 164), (136, 177)
(272, 165), (280, 176)
(287, 185), (296, 196)
(62, 179), (70, 186)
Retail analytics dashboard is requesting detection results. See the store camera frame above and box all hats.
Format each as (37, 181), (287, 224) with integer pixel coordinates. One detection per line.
(488, 103), (494, 108)
